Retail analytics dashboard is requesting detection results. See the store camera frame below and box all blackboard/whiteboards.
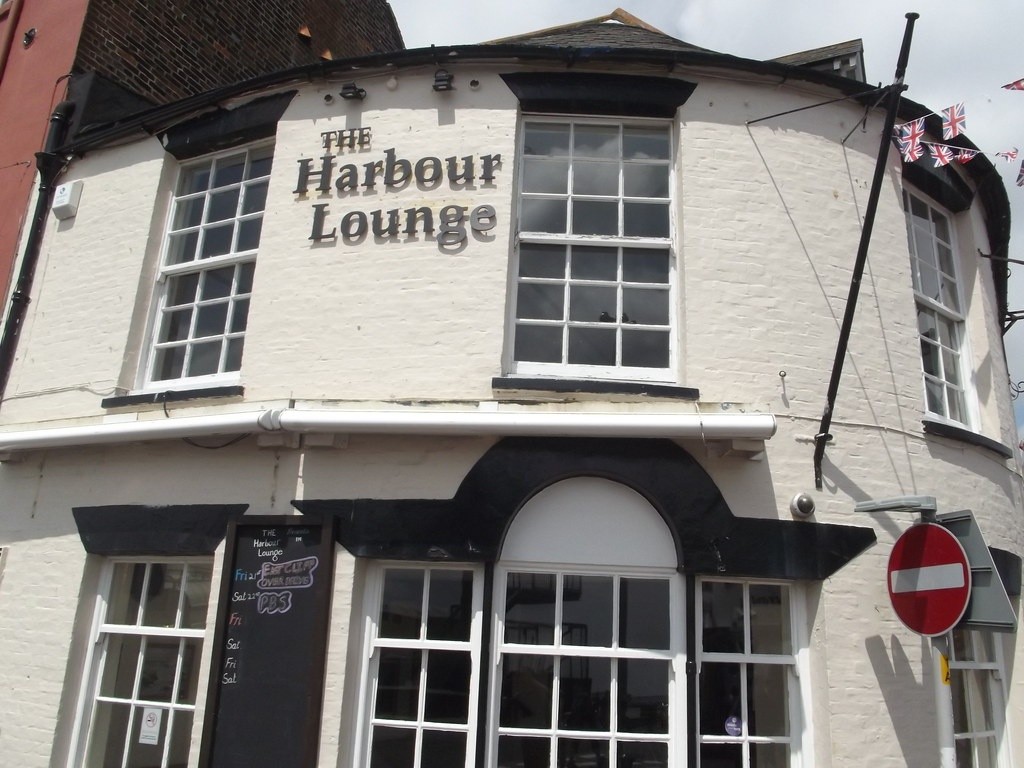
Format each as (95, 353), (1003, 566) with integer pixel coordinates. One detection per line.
(198, 514), (339, 768)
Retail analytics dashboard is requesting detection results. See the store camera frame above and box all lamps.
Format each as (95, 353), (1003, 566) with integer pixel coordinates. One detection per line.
(432, 68), (456, 92)
(339, 80), (366, 101)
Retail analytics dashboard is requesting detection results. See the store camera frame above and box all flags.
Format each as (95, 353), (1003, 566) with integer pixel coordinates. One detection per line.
(942, 102), (966, 140)
(894, 78), (1024, 186)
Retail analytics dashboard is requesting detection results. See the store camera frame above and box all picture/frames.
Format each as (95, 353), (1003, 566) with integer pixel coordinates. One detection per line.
(137, 642), (195, 702)
